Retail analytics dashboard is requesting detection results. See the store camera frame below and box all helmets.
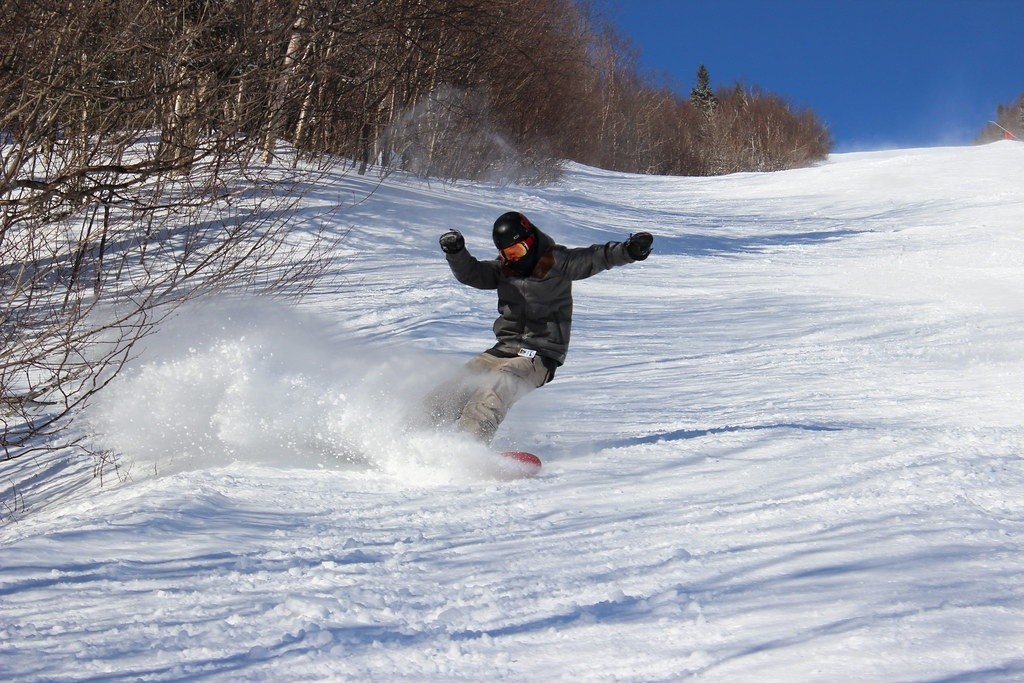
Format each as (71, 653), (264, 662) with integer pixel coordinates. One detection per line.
(492, 211), (535, 250)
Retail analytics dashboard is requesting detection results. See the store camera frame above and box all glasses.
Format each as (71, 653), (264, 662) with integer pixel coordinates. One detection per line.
(497, 234), (536, 262)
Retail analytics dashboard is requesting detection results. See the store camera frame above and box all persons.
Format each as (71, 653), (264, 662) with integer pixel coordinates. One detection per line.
(400, 210), (653, 452)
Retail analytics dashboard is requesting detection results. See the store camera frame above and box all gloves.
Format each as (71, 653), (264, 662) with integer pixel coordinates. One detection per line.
(625, 230), (654, 261)
(439, 228), (464, 254)
